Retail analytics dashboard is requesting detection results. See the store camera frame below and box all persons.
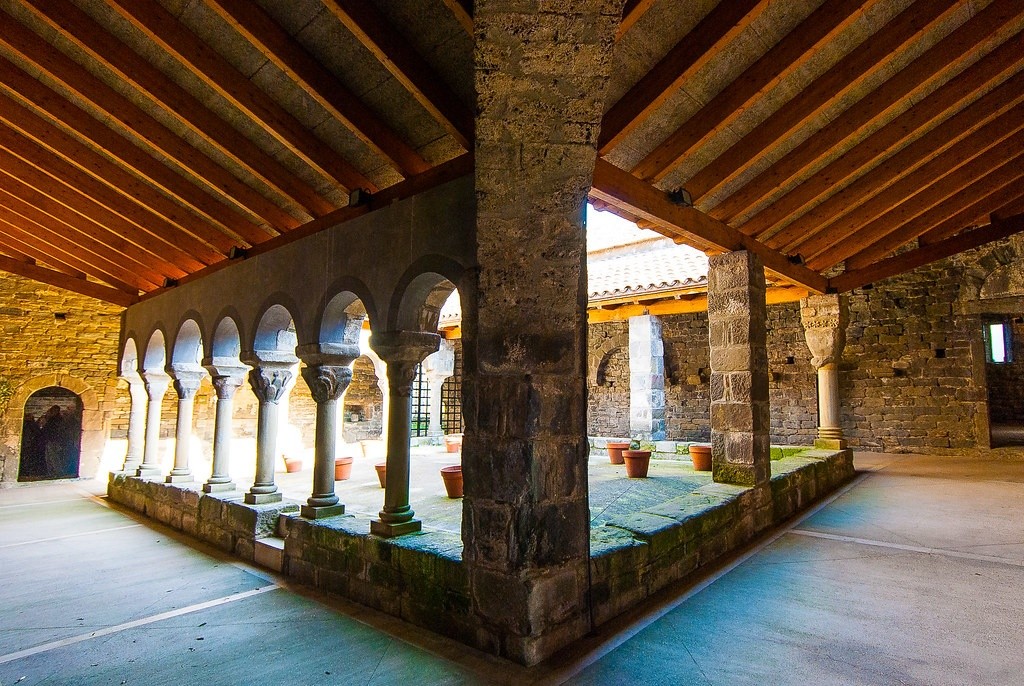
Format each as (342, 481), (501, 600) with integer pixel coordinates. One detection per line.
(42, 405), (65, 480)
(19, 406), (76, 477)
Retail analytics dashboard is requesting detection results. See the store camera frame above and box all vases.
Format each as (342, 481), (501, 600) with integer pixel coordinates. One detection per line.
(375, 462), (386, 488)
(445, 435), (460, 453)
(622, 450), (651, 478)
(606, 443), (629, 464)
(335, 457), (353, 480)
(440, 465), (463, 499)
(689, 445), (713, 471)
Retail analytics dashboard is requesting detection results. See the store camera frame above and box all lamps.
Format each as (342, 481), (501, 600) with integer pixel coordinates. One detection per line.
(228, 245), (246, 259)
(348, 188), (375, 207)
(668, 187), (693, 208)
(788, 253), (805, 265)
(163, 278), (178, 287)
